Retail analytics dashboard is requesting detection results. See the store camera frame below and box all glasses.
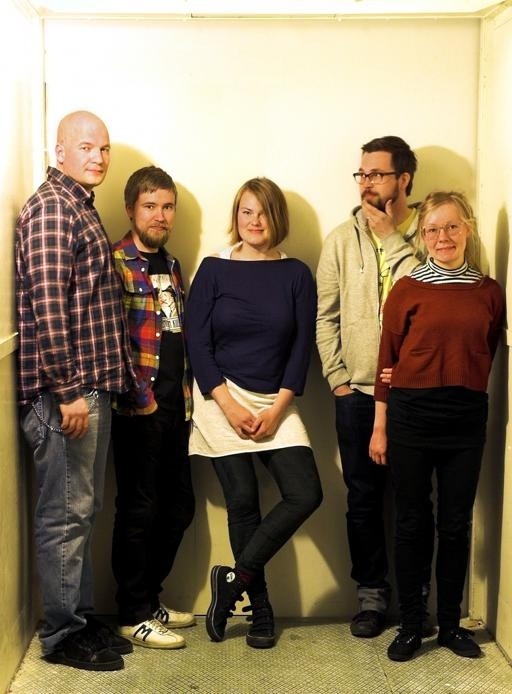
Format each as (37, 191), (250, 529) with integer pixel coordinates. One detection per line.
(354, 172), (399, 184)
(423, 222), (465, 240)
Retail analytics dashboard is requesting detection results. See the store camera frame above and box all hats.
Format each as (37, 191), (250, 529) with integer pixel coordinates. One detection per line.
(162, 284), (175, 293)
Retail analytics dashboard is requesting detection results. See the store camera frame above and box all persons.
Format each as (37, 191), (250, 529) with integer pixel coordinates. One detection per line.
(368, 192), (506, 663)
(14, 111), (133, 669)
(182, 178), (319, 646)
(316, 136), (439, 637)
(111, 166), (196, 650)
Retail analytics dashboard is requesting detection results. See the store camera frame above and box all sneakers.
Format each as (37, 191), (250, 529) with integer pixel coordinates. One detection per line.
(419, 619), (433, 638)
(150, 603), (196, 628)
(43, 633), (123, 669)
(437, 627), (480, 657)
(206, 566), (244, 641)
(349, 610), (383, 637)
(77, 617), (132, 654)
(241, 591), (276, 647)
(117, 618), (186, 649)
(388, 628), (423, 660)
